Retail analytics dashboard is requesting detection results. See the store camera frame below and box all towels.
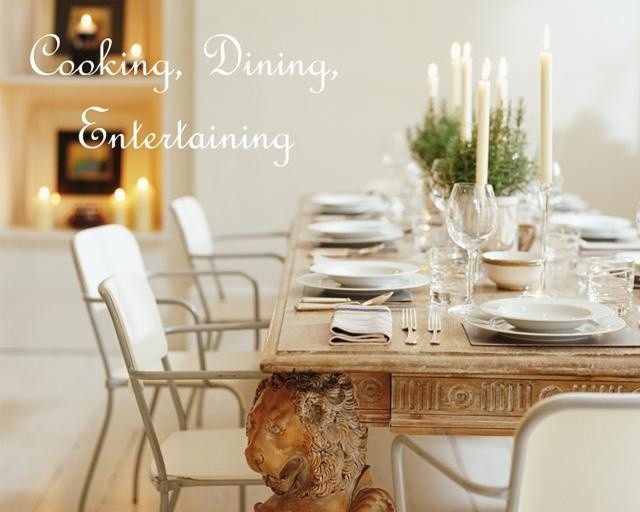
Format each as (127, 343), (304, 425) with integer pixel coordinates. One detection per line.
(328, 302), (394, 348)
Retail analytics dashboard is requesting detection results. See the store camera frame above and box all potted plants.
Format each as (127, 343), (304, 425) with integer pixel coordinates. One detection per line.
(438, 100), (539, 253)
(403, 97), (466, 214)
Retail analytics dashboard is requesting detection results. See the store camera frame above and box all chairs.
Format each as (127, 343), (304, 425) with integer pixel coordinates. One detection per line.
(388, 384), (640, 509)
(97, 270), (299, 511)
(168, 194), (294, 349)
(71, 220), (259, 509)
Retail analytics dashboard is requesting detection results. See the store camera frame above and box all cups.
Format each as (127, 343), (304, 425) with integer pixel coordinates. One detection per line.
(412, 156), (581, 315)
(589, 257), (635, 316)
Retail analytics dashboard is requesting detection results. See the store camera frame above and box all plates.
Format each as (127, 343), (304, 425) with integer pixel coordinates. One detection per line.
(303, 189), (403, 247)
(296, 272), (432, 293)
(464, 312), (628, 344)
(556, 229), (638, 240)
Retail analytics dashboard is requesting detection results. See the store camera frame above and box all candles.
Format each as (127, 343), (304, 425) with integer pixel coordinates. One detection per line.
(124, 43), (147, 66)
(423, 40), (565, 195)
(76, 11), (99, 33)
(29, 178), (155, 238)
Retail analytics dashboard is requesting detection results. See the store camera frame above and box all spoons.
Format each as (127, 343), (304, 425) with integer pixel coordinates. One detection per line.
(295, 290), (395, 311)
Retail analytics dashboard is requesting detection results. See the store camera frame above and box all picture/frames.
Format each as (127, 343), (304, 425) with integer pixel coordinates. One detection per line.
(54, 128), (123, 197)
(50, 0), (124, 58)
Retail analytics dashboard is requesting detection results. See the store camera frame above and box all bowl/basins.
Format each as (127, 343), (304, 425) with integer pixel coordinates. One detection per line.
(565, 214), (630, 235)
(479, 297), (594, 332)
(309, 260), (421, 288)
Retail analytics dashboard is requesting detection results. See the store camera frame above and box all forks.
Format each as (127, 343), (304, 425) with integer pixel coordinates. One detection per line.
(400, 307), (418, 346)
(427, 311), (443, 346)
